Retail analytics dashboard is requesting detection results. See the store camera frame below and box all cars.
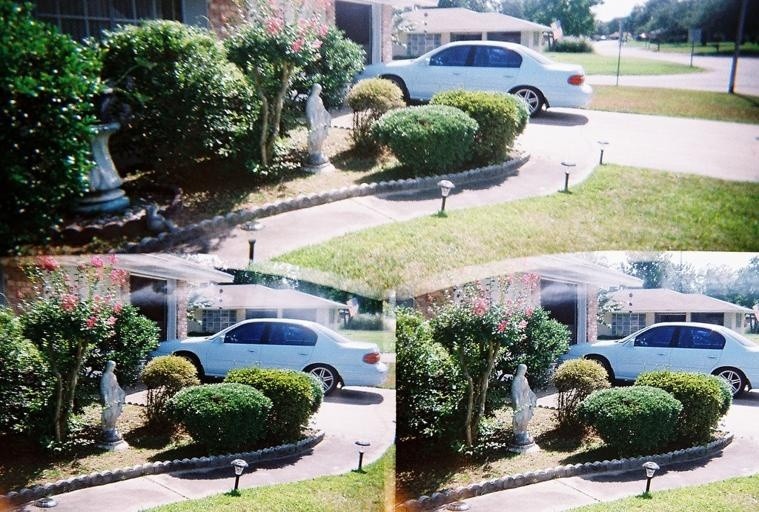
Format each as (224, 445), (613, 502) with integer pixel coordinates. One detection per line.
(555, 322), (759, 399)
(148, 317), (389, 397)
(352, 39), (594, 118)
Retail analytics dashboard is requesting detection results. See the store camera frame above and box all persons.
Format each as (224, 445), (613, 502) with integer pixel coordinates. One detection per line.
(100, 360), (125, 430)
(511, 364), (537, 434)
(305, 83), (331, 165)
